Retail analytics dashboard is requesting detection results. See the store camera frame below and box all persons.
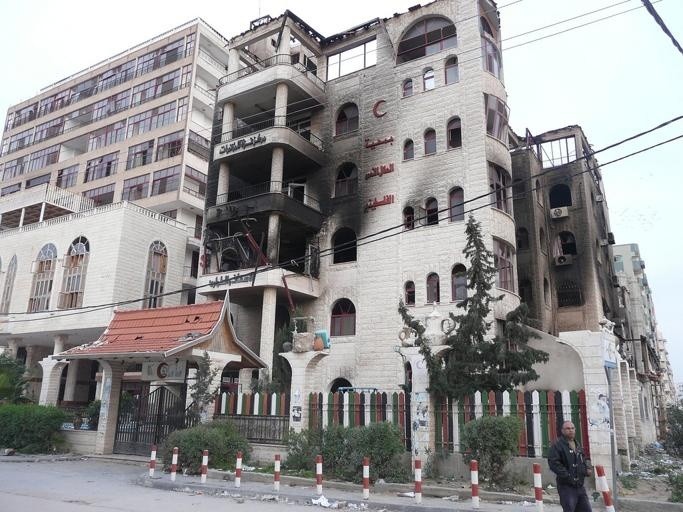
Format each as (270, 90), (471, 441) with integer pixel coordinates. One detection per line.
(548, 420), (594, 512)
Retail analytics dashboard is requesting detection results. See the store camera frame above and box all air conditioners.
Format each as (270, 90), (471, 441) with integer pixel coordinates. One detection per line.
(555, 254), (573, 266)
(550, 206), (569, 219)
